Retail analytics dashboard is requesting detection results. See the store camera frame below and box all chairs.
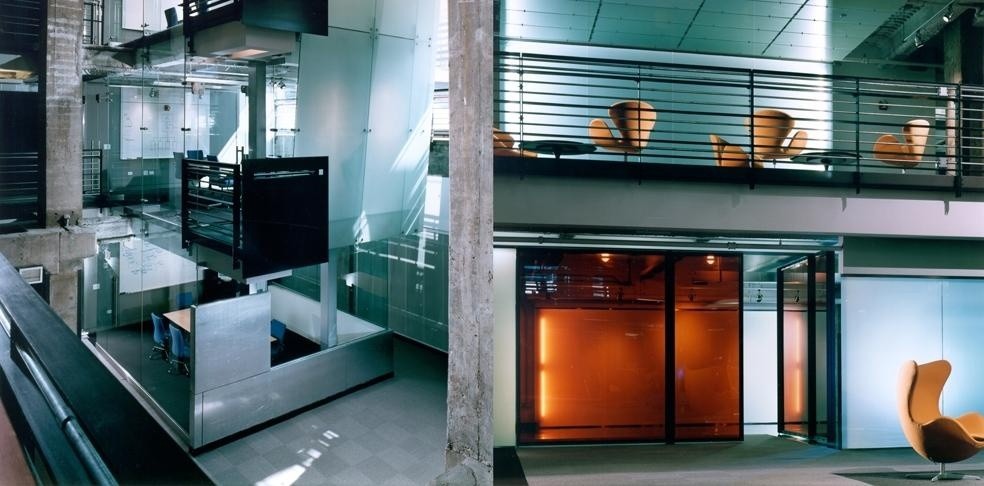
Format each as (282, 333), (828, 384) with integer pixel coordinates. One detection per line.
(494, 128), (540, 157)
(180, 289), (198, 309)
(588, 100), (657, 161)
(870, 119), (930, 173)
(168, 323), (190, 376)
(150, 311), (171, 360)
(747, 110), (809, 168)
(162, 6), (184, 29)
(204, 153), (230, 192)
(172, 152), (199, 185)
(269, 318), (285, 356)
(708, 135), (763, 168)
(900, 359), (983, 486)
(185, 148), (206, 161)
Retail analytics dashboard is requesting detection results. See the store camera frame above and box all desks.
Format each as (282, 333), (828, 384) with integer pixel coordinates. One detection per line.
(788, 153), (863, 170)
(162, 305), (277, 344)
(516, 139), (597, 160)
